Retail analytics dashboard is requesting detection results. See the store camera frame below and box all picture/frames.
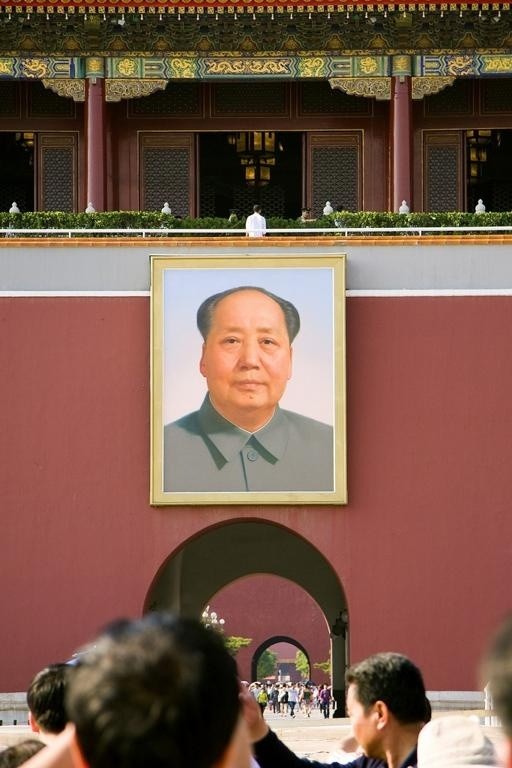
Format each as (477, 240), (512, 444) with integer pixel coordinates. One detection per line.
(150, 253), (348, 506)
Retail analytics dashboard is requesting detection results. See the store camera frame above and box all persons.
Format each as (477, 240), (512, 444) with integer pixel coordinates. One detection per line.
(164, 286), (334, 491)
(240, 679), (332, 719)
(331, 201), (347, 230)
(294, 206), (319, 220)
(407, 714), (496, 767)
(238, 652), (432, 768)
(25, 662), (71, 745)
(474, 616), (511, 767)
(245, 205), (268, 238)
(83, 617), (133, 658)
(0, 734), (45, 767)
(7, 610), (251, 767)
